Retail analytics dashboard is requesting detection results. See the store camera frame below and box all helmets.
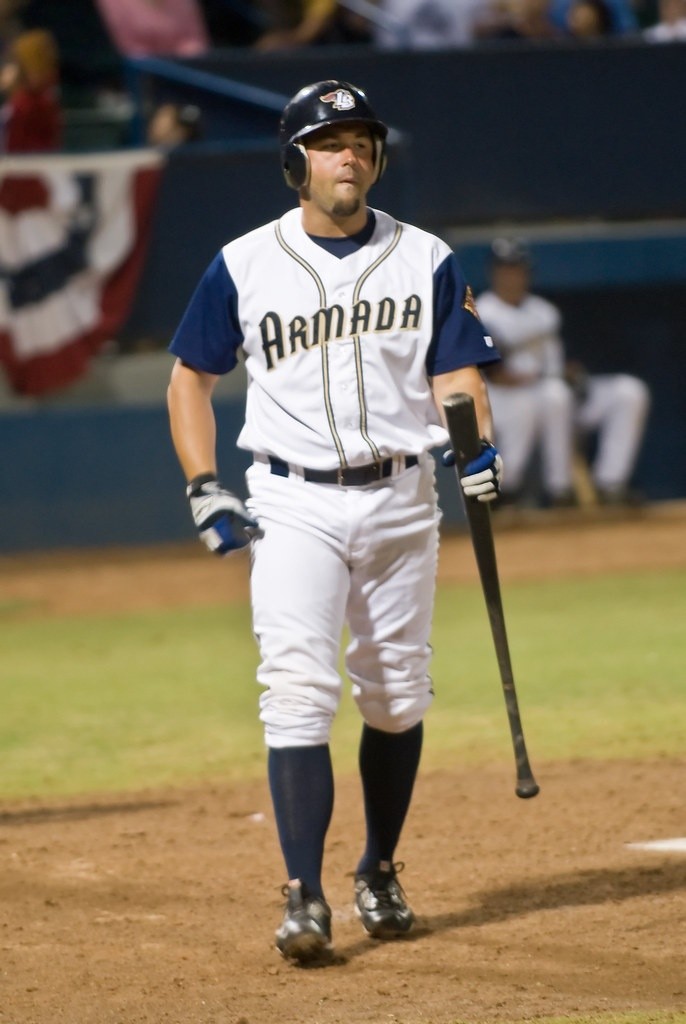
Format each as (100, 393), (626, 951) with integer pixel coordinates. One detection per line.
(279, 80), (387, 190)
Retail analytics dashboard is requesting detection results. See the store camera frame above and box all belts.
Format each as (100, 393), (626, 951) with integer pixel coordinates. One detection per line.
(268, 455), (417, 486)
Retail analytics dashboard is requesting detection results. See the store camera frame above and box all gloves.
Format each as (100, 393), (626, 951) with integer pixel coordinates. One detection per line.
(442, 435), (503, 503)
(186, 474), (258, 555)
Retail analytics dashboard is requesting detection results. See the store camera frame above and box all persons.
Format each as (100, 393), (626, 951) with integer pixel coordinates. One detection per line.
(0, 0), (686, 511)
(168, 80), (505, 958)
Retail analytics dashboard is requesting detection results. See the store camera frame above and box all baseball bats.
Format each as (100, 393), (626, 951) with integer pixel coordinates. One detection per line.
(442, 390), (541, 801)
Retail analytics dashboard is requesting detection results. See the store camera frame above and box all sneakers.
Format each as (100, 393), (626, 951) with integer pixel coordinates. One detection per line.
(274, 878), (334, 966)
(344, 854), (413, 938)
